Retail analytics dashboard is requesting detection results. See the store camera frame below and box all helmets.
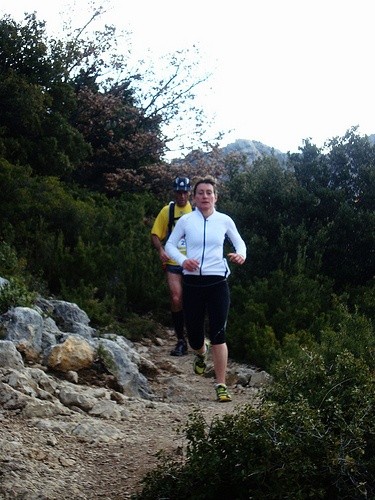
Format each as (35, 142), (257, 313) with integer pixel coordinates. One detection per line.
(173, 177), (190, 192)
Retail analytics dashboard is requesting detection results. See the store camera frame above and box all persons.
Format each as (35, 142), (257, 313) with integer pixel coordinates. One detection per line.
(151, 177), (198, 356)
(164, 176), (247, 402)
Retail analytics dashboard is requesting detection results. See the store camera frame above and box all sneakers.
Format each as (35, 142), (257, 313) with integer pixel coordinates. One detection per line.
(192, 343), (208, 374)
(216, 385), (232, 401)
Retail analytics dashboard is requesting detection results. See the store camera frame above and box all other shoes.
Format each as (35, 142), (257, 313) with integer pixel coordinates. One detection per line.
(170, 341), (187, 355)
